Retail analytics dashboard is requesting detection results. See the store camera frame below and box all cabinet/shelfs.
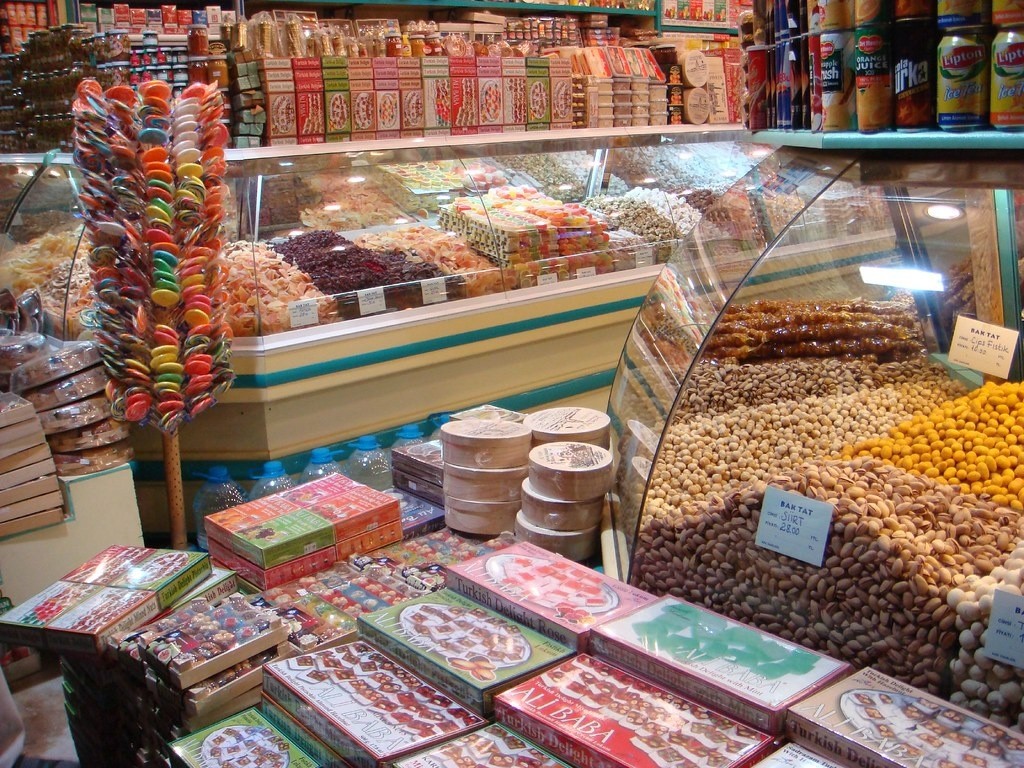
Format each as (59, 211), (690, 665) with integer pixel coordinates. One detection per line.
(325, 0), (739, 37)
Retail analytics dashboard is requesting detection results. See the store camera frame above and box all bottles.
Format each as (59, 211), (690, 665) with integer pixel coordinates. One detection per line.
(342, 435), (394, 492)
(298, 447), (349, 486)
(249, 461), (298, 501)
(427, 414), (453, 441)
(387, 422), (428, 457)
(192, 465), (250, 552)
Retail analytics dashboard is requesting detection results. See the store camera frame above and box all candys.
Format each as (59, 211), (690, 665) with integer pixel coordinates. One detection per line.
(69, 74), (235, 431)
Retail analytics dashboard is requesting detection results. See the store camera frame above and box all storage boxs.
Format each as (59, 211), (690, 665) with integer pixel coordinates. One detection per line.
(0, 0), (1024, 768)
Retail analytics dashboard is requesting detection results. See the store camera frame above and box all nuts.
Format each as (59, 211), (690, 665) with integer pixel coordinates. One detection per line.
(612, 354), (1024, 735)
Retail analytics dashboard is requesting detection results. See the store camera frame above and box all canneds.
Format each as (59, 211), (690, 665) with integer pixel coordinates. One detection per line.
(504, 16), (578, 47)
(385, 33), (442, 57)
(0, 21), (232, 154)
(743, 0), (1024, 132)
